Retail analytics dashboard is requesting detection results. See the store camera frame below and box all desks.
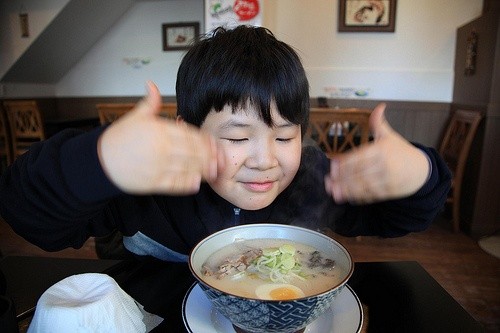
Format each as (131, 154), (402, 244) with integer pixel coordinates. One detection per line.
(96, 103), (371, 159)
(0, 255), (484, 333)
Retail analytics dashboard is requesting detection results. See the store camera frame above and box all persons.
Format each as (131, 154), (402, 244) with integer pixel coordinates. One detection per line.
(0, 22), (452, 264)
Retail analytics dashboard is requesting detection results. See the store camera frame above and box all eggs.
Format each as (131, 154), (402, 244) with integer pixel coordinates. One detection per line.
(254, 283), (305, 300)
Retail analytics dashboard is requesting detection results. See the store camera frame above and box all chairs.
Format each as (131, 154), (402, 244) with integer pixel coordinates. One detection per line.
(439, 110), (480, 231)
(4, 101), (45, 162)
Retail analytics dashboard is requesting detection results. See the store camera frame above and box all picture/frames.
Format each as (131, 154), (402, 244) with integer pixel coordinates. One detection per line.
(162, 21), (199, 52)
(338, 0), (396, 32)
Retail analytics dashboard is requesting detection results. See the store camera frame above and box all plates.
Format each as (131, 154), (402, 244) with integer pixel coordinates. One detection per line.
(181, 278), (364, 333)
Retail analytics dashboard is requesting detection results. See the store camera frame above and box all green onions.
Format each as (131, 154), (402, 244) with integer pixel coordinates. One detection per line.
(248, 245), (315, 282)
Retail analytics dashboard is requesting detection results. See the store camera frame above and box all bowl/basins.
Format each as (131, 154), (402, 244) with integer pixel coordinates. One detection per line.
(187, 223), (354, 333)
